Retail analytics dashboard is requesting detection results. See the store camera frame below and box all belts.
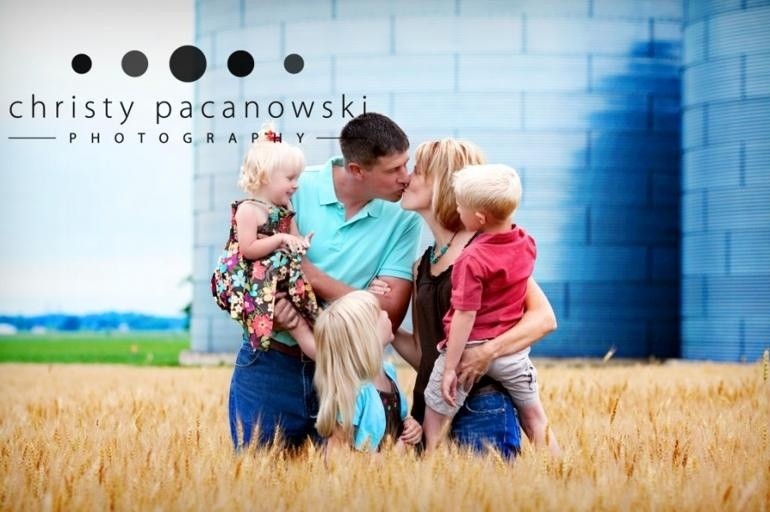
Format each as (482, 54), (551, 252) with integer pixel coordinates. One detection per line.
(472, 381), (505, 397)
(270, 340), (315, 362)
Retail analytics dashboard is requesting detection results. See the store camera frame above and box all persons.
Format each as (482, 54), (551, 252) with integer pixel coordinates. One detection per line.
(211, 125), (327, 362)
(368, 137), (557, 468)
(228, 112), (423, 457)
(423, 165), (559, 466)
(313, 290), (423, 472)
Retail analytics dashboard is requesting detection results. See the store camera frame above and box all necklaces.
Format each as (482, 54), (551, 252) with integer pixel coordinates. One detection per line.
(429, 227), (460, 264)
